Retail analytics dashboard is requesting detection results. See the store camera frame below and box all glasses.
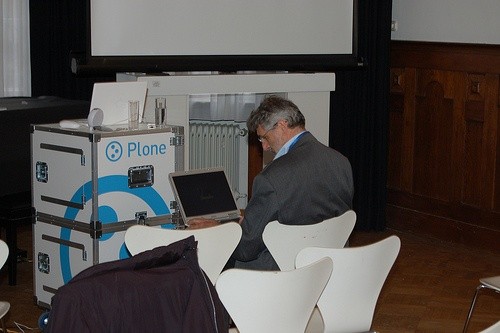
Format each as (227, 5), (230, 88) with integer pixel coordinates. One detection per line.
(256, 120), (288, 142)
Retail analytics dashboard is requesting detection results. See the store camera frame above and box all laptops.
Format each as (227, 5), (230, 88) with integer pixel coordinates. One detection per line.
(72, 81), (148, 127)
(168, 168), (243, 228)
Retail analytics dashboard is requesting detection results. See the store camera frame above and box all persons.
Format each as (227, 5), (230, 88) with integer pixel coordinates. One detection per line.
(185, 96), (354, 328)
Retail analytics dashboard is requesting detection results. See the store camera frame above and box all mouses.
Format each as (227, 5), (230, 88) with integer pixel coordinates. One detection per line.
(60, 120), (80, 129)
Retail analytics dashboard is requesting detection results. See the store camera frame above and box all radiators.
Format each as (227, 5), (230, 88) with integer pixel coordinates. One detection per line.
(189, 119), (249, 201)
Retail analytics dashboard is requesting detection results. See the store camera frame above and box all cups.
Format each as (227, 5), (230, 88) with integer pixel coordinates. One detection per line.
(128, 101), (139, 128)
(156, 98), (166, 108)
(155, 108), (167, 127)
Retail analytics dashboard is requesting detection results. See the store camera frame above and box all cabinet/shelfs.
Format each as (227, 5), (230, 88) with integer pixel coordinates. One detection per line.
(30, 120), (184, 309)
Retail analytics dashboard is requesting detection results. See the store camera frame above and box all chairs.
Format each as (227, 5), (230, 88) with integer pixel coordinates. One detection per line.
(462, 276), (500, 333)
(43, 210), (401, 333)
